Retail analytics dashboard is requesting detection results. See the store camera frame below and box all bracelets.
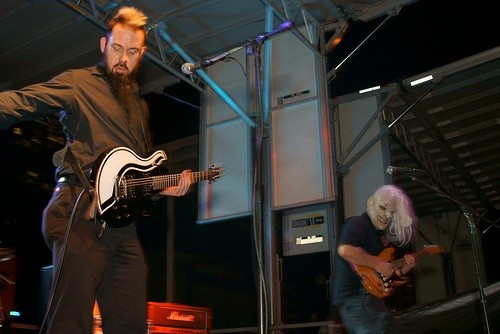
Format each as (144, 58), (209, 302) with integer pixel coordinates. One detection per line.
(398, 267), (404, 276)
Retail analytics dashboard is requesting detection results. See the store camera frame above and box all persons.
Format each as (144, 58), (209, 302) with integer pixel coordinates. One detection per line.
(1, 6), (192, 334)
(331, 184), (417, 334)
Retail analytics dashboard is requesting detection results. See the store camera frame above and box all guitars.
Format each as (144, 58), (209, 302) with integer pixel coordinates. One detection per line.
(351, 244), (441, 298)
(90, 147), (224, 228)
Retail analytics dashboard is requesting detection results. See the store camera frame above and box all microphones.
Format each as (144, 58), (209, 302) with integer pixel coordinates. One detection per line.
(386, 166), (417, 174)
(181, 60), (213, 74)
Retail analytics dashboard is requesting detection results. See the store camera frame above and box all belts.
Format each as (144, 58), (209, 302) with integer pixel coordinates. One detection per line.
(58, 174), (95, 185)
